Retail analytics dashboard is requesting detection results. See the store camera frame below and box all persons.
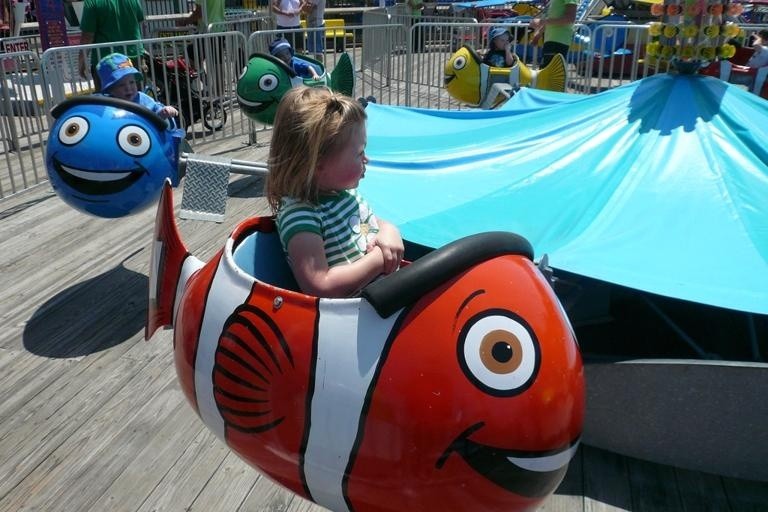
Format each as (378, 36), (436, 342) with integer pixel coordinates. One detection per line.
(267, 35), (321, 83)
(301, 0), (327, 66)
(743, 25), (768, 98)
(271, 0), (304, 53)
(400, 0), (426, 53)
(94, 52), (181, 121)
(74, 0), (148, 91)
(528, 1), (580, 70)
(475, 28), (516, 69)
(265, 81), (406, 299)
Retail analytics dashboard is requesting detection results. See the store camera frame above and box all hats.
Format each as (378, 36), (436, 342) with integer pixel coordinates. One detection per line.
(96, 53), (144, 91)
(488, 24), (514, 49)
(268, 36), (295, 59)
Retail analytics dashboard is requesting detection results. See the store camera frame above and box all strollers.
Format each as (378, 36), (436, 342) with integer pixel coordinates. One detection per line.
(145, 44), (228, 135)
